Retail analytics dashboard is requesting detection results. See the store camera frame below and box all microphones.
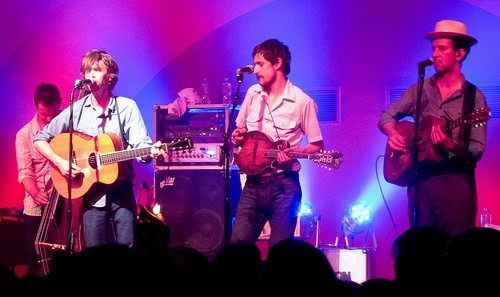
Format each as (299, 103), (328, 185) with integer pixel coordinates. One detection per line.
(418, 57), (435, 67)
(79, 79), (94, 85)
(236, 64), (254, 74)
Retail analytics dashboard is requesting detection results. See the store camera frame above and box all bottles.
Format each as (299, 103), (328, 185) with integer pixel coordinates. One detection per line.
(201, 78), (210, 105)
(222, 78), (231, 105)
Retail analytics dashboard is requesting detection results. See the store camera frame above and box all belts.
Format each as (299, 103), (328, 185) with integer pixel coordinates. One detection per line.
(247, 172), (285, 182)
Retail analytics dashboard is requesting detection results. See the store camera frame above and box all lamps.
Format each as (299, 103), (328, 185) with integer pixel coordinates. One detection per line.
(321, 200), (377, 250)
(298, 199), (321, 247)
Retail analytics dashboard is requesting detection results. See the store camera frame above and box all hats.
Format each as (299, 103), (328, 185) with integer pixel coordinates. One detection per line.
(424, 19), (478, 45)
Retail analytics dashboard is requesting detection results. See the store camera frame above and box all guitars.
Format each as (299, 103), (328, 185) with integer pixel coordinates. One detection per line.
(235, 130), (343, 175)
(383, 107), (492, 187)
(49, 131), (194, 200)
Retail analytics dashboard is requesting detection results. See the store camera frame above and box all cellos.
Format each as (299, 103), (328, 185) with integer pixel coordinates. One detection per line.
(34, 87), (87, 275)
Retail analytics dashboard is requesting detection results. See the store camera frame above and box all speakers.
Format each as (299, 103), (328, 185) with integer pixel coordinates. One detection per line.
(318, 246), (376, 284)
(154, 168), (243, 261)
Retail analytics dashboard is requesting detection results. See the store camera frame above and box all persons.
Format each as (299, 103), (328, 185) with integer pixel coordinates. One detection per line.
(376, 20), (487, 232)
(15, 83), (67, 243)
(0, 225), (500, 297)
(32, 48), (167, 248)
(231, 38), (325, 252)
